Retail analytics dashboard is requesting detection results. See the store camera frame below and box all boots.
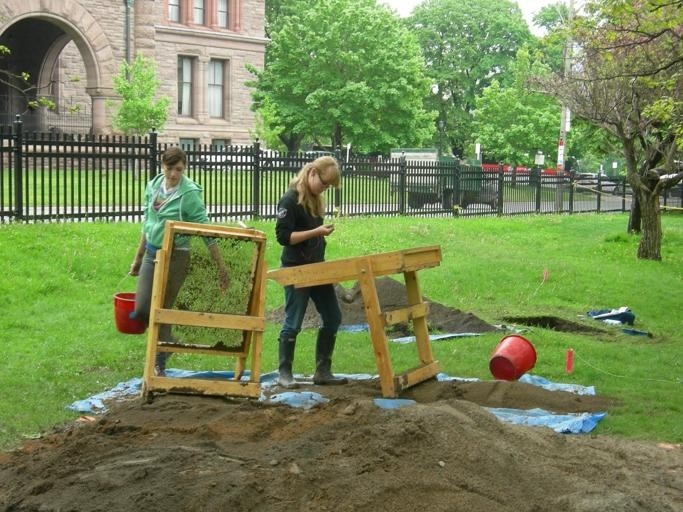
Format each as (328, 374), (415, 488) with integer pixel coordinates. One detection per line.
(313, 326), (347, 387)
(274, 332), (302, 392)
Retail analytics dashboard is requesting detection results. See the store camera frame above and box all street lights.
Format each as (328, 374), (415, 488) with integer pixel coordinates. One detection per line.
(438, 120), (444, 155)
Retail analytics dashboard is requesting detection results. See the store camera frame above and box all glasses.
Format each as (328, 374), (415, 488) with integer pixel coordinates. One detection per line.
(318, 172), (331, 189)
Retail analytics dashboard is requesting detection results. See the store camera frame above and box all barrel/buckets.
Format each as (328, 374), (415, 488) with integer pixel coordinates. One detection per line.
(489, 334), (536, 381)
(113, 272), (145, 334)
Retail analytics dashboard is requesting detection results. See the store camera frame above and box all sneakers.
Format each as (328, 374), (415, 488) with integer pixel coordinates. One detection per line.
(153, 365), (166, 378)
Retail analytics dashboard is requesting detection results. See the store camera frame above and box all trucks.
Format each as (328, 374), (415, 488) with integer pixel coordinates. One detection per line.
(390, 153), (498, 209)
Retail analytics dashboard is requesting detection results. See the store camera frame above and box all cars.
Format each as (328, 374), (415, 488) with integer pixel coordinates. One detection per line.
(573, 173), (619, 195)
(201, 146), (279, 170)
(306, 145), (332, 158)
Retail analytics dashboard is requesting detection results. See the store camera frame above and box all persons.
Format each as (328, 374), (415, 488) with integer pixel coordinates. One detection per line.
(274, 156), (346, 389)
(130, 147), (230, 376)
